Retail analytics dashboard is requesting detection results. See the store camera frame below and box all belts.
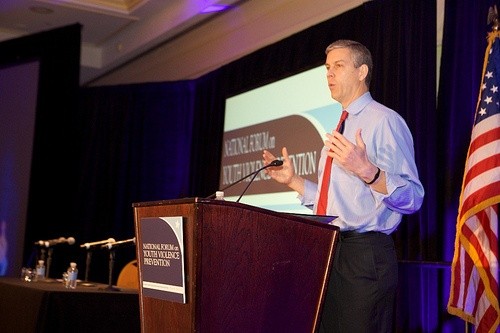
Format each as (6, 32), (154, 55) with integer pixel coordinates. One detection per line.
(341, 230), (375, 237)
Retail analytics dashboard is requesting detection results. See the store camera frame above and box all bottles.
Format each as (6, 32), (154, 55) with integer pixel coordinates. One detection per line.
(66, 263), (78, 289)
(215, 191), (225, 201)
(36, 261), (45, 281)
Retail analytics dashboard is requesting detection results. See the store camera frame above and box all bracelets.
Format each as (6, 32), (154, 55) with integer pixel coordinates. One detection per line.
(364, 167), (380, 185)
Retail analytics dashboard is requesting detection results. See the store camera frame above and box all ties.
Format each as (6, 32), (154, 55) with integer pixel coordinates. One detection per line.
(316, 111), (349, 216)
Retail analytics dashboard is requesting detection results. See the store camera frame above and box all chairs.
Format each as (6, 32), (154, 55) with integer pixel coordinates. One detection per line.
(116, 260), (139, 290)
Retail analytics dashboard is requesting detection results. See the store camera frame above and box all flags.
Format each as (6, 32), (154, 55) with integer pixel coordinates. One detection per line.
(447, 31), (500, 333)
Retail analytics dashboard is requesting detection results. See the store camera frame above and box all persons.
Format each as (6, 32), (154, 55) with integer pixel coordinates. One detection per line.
(263, 40), (424, 333)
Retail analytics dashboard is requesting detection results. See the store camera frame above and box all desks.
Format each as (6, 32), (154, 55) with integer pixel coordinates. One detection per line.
(0, 278), (140, 333)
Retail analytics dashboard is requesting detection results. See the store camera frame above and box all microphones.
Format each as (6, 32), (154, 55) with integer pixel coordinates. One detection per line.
(101, 237), (136, 249)
(79, 238), (115, 250)
(42, 237), (75, 247)
(33, 237), (67, 246)
(207, 160), (283, 198)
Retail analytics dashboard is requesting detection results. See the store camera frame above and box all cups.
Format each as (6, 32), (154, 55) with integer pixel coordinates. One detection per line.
(62, 273), (68, 285)
(23, 268), (35, 283)
